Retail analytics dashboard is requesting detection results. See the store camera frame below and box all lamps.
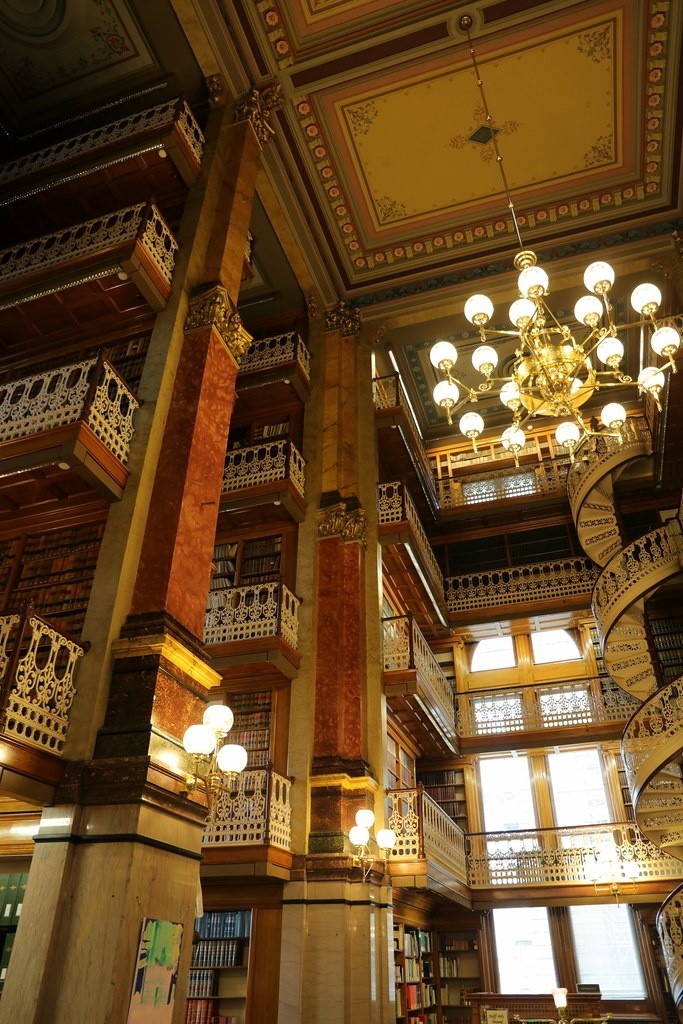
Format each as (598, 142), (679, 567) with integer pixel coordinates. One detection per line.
(183, 705), (248, 821)
(429, 16), (680, 466)
(552, 988), (569, 1024)
(348, 809), (397, 885)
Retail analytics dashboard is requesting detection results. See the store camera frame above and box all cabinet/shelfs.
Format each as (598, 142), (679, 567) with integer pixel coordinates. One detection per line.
(393, 923), (485, 1024)
(184, 907), (255, 1024)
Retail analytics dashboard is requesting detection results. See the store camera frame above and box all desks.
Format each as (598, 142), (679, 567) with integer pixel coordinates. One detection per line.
(465, 991), (683, 1024)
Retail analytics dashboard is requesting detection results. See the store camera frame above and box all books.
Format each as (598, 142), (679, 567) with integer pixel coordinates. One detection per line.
(0, 524), (104, 678)
(185, 911), (250, 1024)
(81, 336), (150, 430)
(387, 735), (459, 839)
(222, 692), (271, 766)
(254, 421), (288, 439)
(394, 924), (479, 1024)
(209, 537), (281, 619)
